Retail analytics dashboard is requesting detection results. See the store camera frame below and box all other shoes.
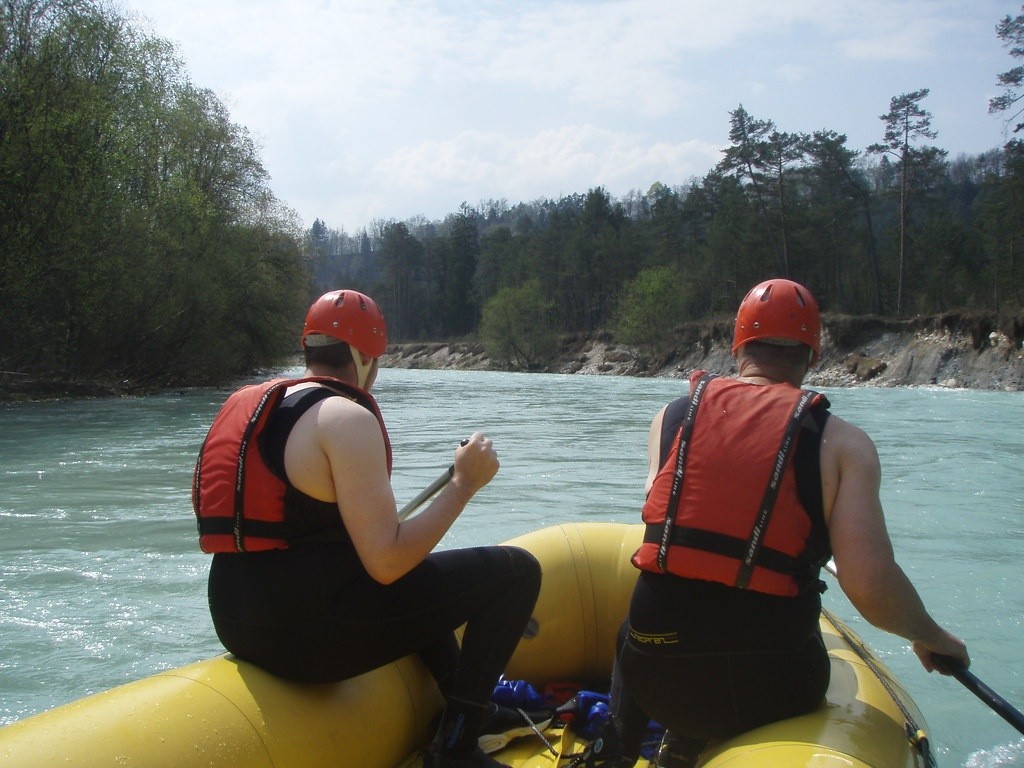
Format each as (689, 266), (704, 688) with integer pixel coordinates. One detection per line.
(477, 703), (553, 756)
(423, 743), (512, 768)
(657, 730), (701, 767)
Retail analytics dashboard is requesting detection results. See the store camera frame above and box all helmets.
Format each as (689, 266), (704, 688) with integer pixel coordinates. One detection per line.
(732, 279), (821, 367)
(302, 291), (387, 358)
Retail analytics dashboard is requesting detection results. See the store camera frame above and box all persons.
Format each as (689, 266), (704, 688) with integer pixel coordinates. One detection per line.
(611, 281), (971, 757)
(193, 290), (543, 768)
